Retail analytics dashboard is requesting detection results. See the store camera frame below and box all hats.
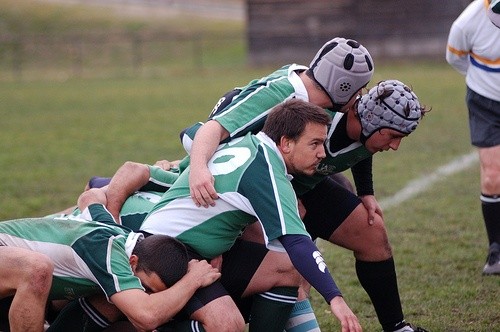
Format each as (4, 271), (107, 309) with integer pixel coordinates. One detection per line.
(309, 37), (374, 106)
(358, 80), (422, 138)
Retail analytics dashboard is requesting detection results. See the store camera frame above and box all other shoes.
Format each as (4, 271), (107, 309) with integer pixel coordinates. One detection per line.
(481, 252), (500, 274)
(383, 318), (429, 332)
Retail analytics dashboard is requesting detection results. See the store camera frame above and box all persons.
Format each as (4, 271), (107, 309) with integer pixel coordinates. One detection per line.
(186, 36), (378, 332)
(289, 78), (436, 332)
(0, 99), (364, 332)
(444, 0), (500, 275)
